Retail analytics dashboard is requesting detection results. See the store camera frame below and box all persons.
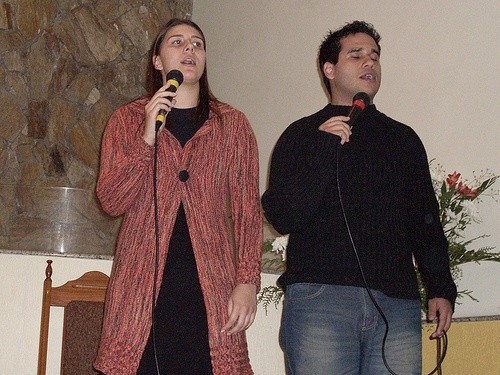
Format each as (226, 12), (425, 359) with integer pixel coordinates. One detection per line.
(260, 21), (457, 375)
(93, 18), (264, 375)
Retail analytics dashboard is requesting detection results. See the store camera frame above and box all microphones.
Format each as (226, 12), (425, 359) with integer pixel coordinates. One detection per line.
(337, 92), (370, 145)
(155, 69), (183, 131)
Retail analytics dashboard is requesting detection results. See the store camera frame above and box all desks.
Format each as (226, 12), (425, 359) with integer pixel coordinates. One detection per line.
(421, 314), (500, 375)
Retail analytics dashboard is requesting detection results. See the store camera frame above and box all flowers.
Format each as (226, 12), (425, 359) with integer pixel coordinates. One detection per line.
(256, 159), (500, 319)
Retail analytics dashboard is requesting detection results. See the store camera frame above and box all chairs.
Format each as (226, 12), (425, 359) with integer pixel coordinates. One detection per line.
(38, 260), (109, 375)
